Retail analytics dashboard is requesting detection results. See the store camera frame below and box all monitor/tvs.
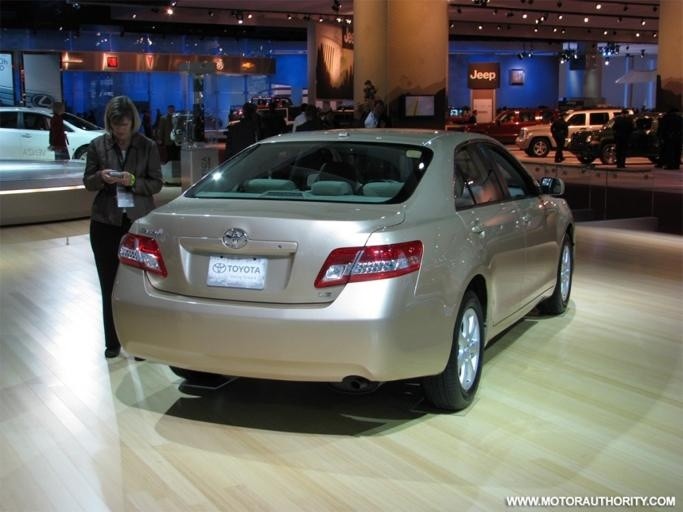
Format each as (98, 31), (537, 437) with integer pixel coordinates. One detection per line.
(459, 108), (463, 115)
(404, 95), (435, 117)
(450, 108), (458, 117)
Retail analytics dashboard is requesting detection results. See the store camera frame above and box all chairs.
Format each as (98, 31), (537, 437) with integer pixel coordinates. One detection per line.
(242, 179), (405, 199)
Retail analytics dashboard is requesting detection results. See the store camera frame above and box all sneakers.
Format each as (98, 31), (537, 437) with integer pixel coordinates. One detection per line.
(104, 345), (120, 358)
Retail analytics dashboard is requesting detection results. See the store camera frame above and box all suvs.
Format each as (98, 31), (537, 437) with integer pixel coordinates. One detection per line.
(467, 109), (549, 143)
(567, 112), (661, 163)
(517, 108), (632, 159)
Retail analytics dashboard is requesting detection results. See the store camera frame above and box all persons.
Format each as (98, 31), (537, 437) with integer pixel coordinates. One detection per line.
(82, 94), (165, 363)
(550, 113), (569, 164)
(612, 105), (683, 170)
(48, 101), (70, 159)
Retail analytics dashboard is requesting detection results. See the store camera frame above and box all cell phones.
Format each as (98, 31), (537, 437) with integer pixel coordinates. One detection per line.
(110, 171), (121, 178)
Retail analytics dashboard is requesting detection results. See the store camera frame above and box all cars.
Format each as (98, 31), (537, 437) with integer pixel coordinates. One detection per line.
(0, 107), (106, 161)
(112, 127), (575, 411)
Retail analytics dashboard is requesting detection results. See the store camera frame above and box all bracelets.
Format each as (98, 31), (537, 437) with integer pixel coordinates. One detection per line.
(130, 173), (136, 187)
